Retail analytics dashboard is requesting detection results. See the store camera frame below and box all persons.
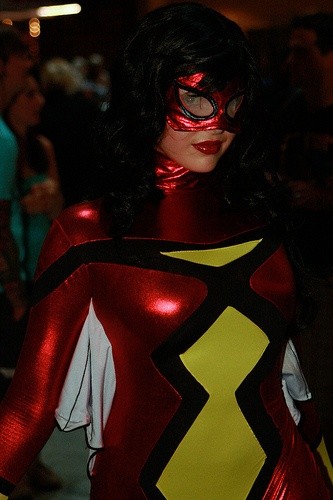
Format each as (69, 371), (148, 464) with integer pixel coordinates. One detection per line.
(0, 28), (34, 385)
(50, 11), (332, 223)
(9, 73), (63, 278)
(0, 4), (333, 499)
